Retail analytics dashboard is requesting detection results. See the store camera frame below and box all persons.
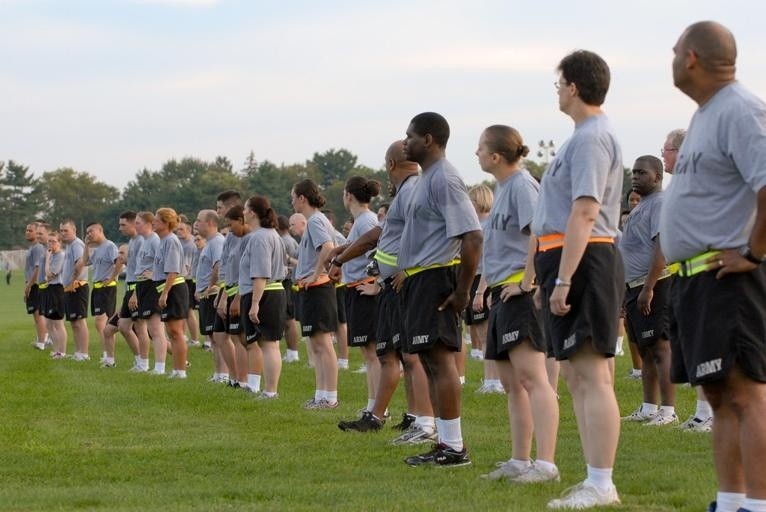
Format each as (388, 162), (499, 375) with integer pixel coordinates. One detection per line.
(287, 113), (559, 484)
(672, 21), (766, 512)
(26, 188), (299, 397)
(616, 128), (714, 433)
(532, 49), (623, 509)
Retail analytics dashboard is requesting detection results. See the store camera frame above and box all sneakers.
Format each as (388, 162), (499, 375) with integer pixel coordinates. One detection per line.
(304, 397), (471, 467)
(31, 332), (210, 380)
(283, 357), (367, 376)
(206, 373), (277, 400)
(620, 402), (714, 433)
(480, 458), (621, 511)
(461, 347), (506, 394)
(614, 350), (644, 382)
